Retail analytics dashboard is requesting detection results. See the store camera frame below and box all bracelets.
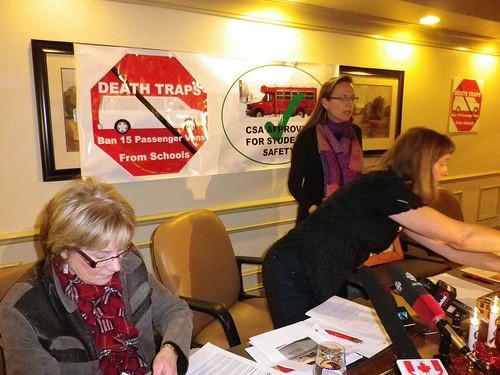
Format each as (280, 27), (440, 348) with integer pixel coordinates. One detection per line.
(160, 343), (180, 357)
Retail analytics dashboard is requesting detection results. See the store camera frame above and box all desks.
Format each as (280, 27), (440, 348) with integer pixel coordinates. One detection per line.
(228, 264), (499, 375)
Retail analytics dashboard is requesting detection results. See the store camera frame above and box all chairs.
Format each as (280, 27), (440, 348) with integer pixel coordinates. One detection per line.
(408, 188), (464, 256)
(151, 211), (275, 348)
(337, 232), (451, 300)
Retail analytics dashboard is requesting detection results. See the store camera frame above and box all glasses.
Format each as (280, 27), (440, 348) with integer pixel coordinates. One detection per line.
(327, 95), (359, 104)
(77, 245), (132, 269)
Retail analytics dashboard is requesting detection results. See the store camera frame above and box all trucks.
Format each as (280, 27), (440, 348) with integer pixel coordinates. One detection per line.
(246, 87), (319, 120)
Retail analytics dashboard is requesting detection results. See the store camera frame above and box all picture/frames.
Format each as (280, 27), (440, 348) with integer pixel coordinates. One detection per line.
(31, 39), (405, 182)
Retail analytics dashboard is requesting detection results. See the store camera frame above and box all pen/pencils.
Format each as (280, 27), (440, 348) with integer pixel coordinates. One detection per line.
(325, 329), (364, 344)
(462, 272), (493, 284)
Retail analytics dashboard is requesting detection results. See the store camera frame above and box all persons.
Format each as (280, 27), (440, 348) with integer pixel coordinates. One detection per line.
(261, 127), (500, 330)
(288, 75), (364, 225)
(0, 175), (193, 375)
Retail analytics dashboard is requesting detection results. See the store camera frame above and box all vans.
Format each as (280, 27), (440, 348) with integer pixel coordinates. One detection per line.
(452, 96), (480, 113)
(97, 95), (206, 135)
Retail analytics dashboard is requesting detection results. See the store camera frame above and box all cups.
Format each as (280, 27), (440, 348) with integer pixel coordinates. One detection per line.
(314, 341), (346, 375)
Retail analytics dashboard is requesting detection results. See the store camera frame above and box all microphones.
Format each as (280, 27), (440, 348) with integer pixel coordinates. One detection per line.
(357, 265), (448, 375)
(415, 276), (474, 315)
(387, 261), (465, 351)
